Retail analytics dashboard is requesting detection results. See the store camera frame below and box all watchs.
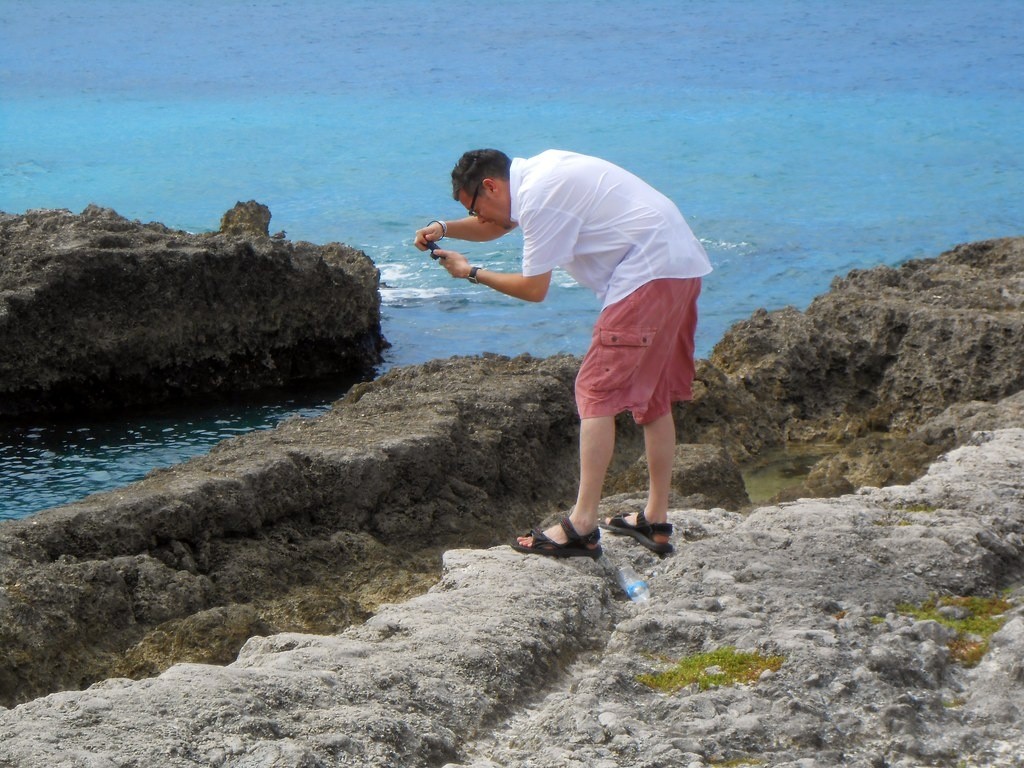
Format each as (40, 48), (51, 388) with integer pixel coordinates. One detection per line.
(468, 266), (481, 284)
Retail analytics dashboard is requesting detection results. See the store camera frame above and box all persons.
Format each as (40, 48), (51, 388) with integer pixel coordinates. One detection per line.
(414, 147), (713, 558)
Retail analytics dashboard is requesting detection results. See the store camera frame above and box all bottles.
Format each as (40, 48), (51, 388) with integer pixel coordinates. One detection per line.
(616, 565), (650, 605)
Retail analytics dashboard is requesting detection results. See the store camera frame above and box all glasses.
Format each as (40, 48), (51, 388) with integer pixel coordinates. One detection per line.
(469, 182), (482, 216)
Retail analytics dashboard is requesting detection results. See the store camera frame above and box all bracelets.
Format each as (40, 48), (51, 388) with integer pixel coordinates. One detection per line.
(426, 220), (448, 241)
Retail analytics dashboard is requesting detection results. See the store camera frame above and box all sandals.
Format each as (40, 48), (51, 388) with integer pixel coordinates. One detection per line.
(600, 510), (673, 552)
(509, 516), (602, 558)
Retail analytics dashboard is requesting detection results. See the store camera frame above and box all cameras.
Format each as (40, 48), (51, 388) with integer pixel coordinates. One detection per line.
(422, 241), (446, 260)
